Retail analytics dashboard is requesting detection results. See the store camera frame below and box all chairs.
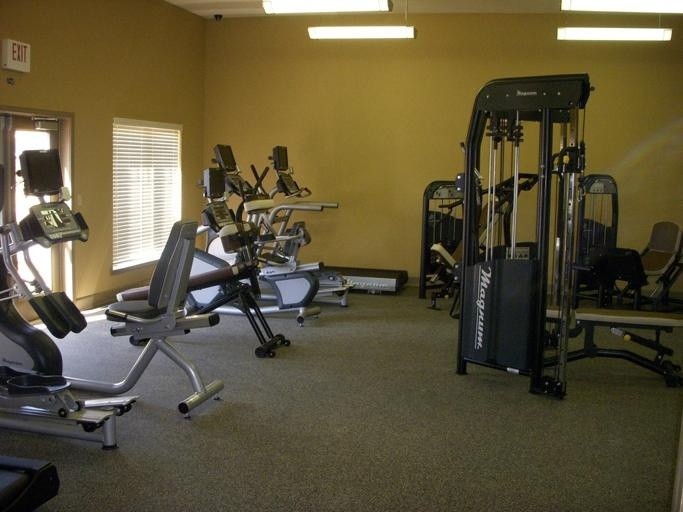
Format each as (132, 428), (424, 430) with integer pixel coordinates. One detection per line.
(105, 220), (222, 412)
(640, 220), (683, 305)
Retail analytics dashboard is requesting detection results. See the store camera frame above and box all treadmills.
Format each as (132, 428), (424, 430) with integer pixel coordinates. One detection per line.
(235, 146), (408, 295)
